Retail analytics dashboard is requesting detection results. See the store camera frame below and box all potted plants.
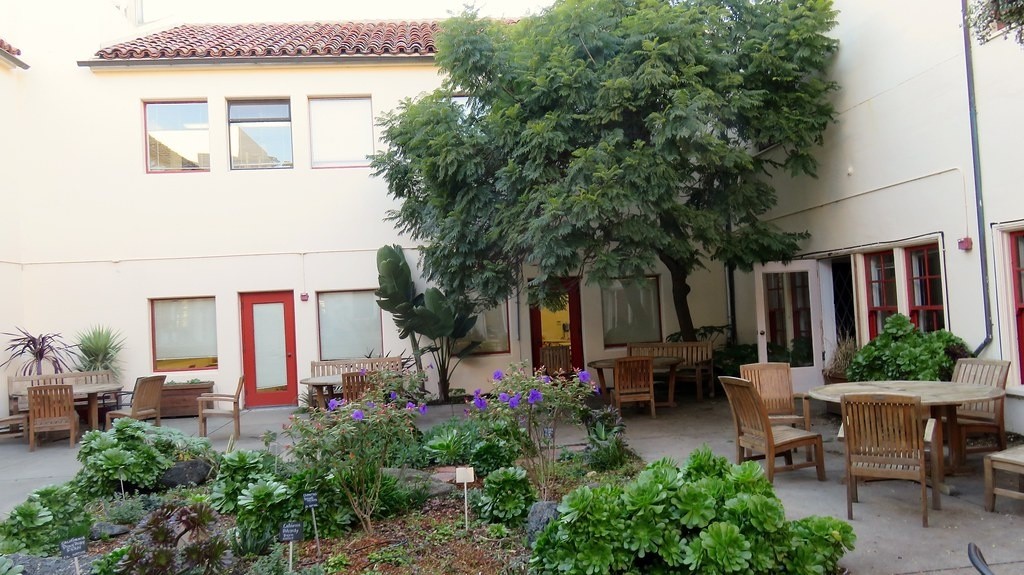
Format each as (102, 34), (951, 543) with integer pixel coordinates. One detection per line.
(160, 378), (214, 419)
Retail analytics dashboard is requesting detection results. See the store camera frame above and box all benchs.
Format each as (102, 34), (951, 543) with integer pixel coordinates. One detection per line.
(311, 357), (404, 398)
(626, 341), (715, 403)
(8, 371), (116, 438)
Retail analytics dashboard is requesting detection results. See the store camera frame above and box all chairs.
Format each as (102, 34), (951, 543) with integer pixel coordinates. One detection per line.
(105, 375), (167, 433)
(26, 385), (80, 452)
(540, 346), (570, 373)
(610, 356), (657, 420)
(840, 392), (942, 528)
(0, 414), (29, 445)
(968, 542), (995, 575)
(739, 362), (813, 462)
(342, 372), (367, 403)
(984, 445), (1024, 512)
(941, 358), (1012, 464)
(195, 377), (244, 441)
(717, 375), (826, 484)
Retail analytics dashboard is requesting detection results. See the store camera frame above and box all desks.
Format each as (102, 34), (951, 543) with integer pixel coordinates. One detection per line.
(300, 375), (371, 411)
(12, 383), (123, 430)
(588, 355), (686, 409)
(808, 381), (1007, 496)
